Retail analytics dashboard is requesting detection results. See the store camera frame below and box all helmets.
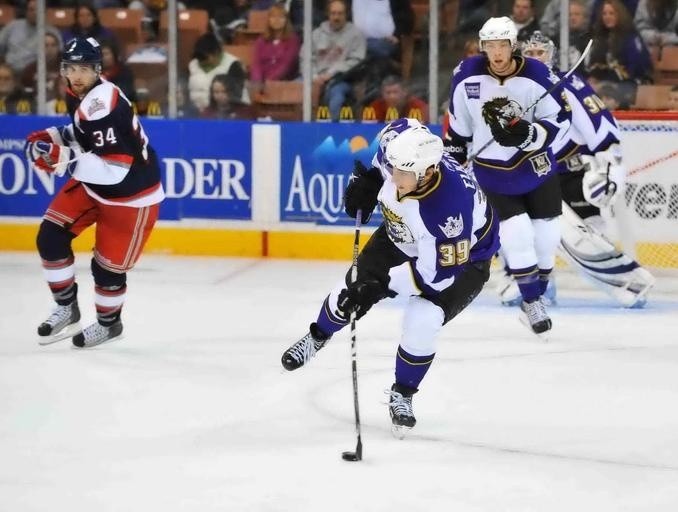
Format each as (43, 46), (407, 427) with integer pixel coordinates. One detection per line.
(478, 16), (518, 52)
(59, 38), (102, 76)
(386, 128), (443, 181)
(522, 30), (554, 68)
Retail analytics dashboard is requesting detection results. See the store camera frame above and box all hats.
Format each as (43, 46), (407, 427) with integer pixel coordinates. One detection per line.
(190, 34), (222, 60)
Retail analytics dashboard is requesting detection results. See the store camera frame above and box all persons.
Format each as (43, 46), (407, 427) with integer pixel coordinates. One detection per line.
(440, 16), (573, 335)
(441, 100), (656, 308)
(282, 116), (500, 441)
(1, 2), (677, 117)
(520, 29), (625, 245)
(25, 34), (168, 346)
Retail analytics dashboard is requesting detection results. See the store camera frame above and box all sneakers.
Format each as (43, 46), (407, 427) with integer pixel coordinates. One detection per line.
(389, 383), (419, 427)
(38, 300), (81, 336)
(282, 323), (331, 371)
(73, 321), (122, 347)
(521, 299), (552, 334)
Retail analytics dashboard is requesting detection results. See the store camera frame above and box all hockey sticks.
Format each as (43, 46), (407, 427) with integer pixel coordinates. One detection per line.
(340, 208), (363, 462)
(461, 40), (593, 162)
(42, 149), (92, 168)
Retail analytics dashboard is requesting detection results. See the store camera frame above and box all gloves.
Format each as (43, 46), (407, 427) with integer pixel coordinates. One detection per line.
(345, 160), (384, 225)
(25, 141), (71, 178)
(444, 139), (467, 165)
(491, 116), (537, 149)
(336, 275), (398, 321)
(27, 126), (65, 146)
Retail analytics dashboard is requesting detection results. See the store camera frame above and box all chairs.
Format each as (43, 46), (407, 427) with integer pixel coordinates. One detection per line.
(224, 43), (256, 65)
(630, 83), (677, 112)
(1, 2), (209, 80)
(252, 75), (322, 124)
(646, 44), (678, 71)
(412, 1), (459, 34)
(237, 6), (269, 35)
